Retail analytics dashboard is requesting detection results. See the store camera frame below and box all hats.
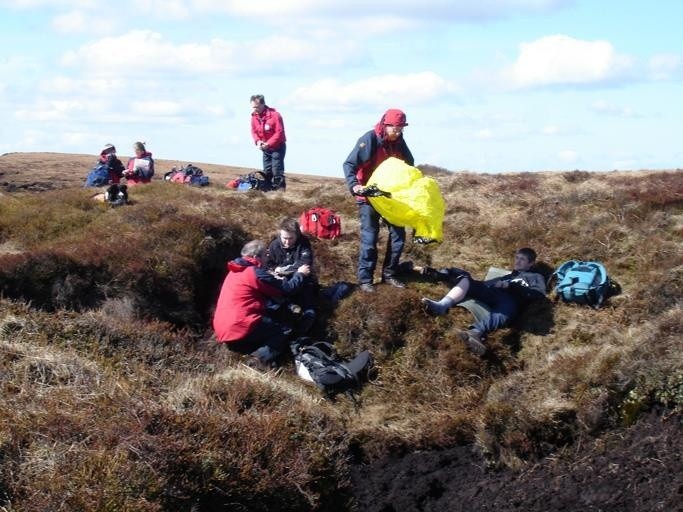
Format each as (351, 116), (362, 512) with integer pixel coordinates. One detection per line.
(381, 109), (408, 127)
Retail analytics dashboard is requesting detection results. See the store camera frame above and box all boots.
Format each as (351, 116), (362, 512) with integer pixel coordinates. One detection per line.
(459, 328), (486, 356)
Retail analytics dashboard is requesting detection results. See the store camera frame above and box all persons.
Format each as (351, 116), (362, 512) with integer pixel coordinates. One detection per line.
(421, 248), (548, 359)
(213, 238), (312, 372)
(97, 142), (129, 186)
(80, 159), (123, 188)
(342, 108), (415, 295)
(263, 216), (318, 348)
(249, 92), (288, 192)
(125, 141), (155, 186)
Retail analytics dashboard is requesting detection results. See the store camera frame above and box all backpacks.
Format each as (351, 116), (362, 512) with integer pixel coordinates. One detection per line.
(545, 259), (620, 310)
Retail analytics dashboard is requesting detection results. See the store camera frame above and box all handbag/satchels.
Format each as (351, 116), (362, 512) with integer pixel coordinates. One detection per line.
(299, 207), (340, 242)
(294, 341), (375, 404)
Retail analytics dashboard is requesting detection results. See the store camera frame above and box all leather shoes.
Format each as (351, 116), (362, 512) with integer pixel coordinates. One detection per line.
(383, 276), (406, 288)
(359, 283), (376, 292)
